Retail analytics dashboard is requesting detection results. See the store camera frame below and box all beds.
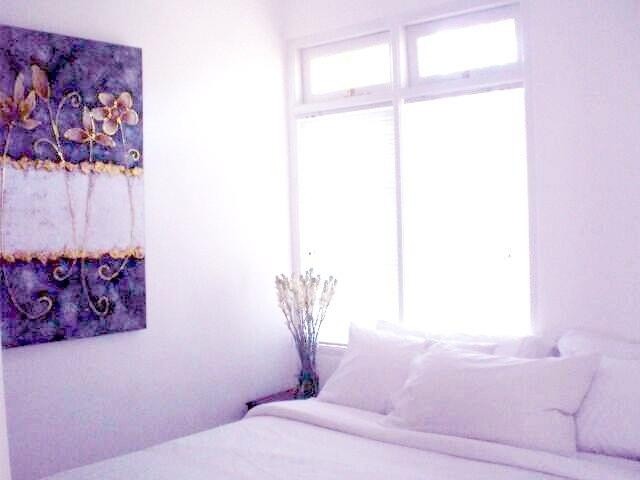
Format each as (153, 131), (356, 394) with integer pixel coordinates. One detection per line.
(42, 336), (640, 480)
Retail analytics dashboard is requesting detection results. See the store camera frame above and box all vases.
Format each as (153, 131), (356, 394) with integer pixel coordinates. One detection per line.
(290, 365), (321, 399)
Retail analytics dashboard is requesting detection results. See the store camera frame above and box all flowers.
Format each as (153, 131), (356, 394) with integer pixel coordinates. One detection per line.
(274, 262), (340, 363)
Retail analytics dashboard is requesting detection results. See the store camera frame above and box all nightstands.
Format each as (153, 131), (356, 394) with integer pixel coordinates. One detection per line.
(247, 385), (310, 411)
